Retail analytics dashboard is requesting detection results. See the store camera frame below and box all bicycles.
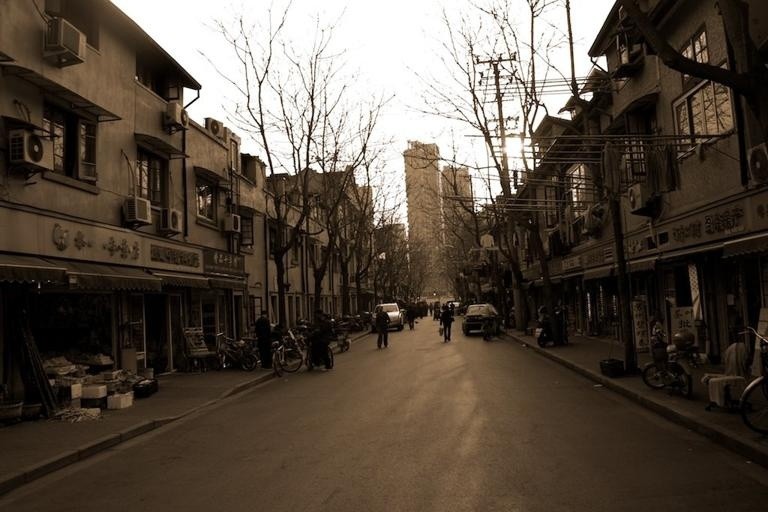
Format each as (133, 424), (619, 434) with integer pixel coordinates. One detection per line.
(215, 313), (364, 378)
(737, 325), (767, 436)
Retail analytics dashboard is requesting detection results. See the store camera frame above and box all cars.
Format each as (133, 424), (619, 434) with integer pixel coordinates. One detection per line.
(462, 304), (499, 335)
(369, 302), (405, 333)
(447, 301), (466, 316)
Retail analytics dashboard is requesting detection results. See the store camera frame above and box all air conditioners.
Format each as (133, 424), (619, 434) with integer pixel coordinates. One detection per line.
(628, 184), (653, 212)
(10, 129), (55, 171)
(746, 143), (768, 187)
(222, 213), (242, 233)
(167, 102), (225, 138)
(125, 196), (183, 234)
(44, 17), (88, 61)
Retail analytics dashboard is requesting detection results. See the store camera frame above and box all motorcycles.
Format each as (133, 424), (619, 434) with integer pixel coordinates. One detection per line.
(533, 304), (568, 348)
(641, 321), (699, 399)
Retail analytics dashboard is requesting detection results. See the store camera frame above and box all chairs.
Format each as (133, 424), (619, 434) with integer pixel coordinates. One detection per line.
(702, 342), (752, 412)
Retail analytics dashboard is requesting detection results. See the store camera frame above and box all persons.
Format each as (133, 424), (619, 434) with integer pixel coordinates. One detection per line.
(428, 301), (455, 321)
(303, 308), (334, 370)
(255, 310), (274, 369)
(439, 306), (453, 342)
(376, 306), (390, 348)
(406, 305), (414, 331)
